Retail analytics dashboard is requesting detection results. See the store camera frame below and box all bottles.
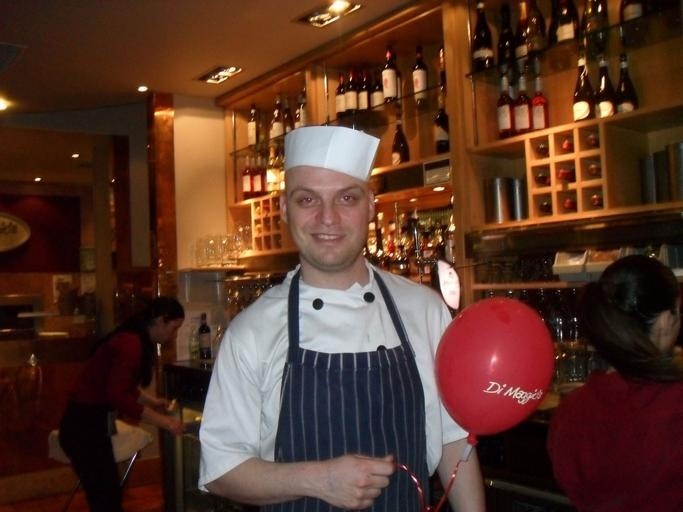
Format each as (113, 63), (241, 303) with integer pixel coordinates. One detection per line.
(481, 252), (614, 386)
(241, 87), (305, 250)
(532, 135), (604, 218)
(188, 326), (199, 360)
(572, 50), (640, 125)
(334, 46), (449, 165)
(360, 196), (456, 278)
(496, 75), (550, 139)
(199, 313), (212, 360)
(470, 2), (680, 84)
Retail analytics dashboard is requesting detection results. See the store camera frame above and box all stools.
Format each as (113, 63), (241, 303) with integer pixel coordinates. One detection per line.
(49, 419), (154, 512)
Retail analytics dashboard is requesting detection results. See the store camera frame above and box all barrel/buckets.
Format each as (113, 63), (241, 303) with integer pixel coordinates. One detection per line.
(482, 175), (525, 223)
(638, 142), (681, 214)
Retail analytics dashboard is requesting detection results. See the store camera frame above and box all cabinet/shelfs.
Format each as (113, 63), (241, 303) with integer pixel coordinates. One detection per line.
(214, 1), (683, 356)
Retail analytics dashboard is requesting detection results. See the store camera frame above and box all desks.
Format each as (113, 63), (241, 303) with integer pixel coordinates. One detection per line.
(0, 324), (105, 479)
(163, 357), (566, 512)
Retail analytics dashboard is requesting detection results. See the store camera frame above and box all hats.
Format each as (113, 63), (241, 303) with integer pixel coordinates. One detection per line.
(284, 125), (380, 181)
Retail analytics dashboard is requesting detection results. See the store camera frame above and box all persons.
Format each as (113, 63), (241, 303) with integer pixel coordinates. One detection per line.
(547, 256), (681, 510)
(58, 294), (184, 512)
(199, 125), (485, 511)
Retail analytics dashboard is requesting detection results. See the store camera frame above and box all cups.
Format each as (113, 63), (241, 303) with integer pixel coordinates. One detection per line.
(195, 234), (239, 266)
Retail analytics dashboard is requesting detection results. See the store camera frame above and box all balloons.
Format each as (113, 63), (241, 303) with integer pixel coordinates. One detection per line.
(435, 296), (555, 445)
